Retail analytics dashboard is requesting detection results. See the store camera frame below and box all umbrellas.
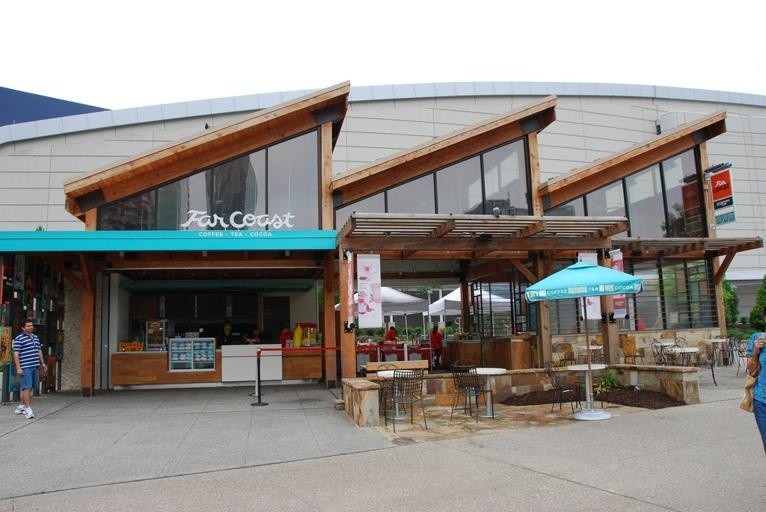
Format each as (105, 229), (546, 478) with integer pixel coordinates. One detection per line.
(525, 262), (645, 411)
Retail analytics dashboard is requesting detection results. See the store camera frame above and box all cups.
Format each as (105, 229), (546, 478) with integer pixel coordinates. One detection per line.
(368, 339), (373, 345)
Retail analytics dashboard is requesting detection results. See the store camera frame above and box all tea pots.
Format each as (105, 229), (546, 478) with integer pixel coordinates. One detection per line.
(412, 339), (419, 348)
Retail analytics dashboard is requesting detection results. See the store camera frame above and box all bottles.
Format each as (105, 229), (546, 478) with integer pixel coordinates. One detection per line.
(293, 322), (303, 347)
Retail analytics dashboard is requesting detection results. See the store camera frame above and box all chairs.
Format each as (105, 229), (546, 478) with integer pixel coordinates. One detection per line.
(545, 359), (583, 412)
(450, 366), (495, 425)
(384, 368), (427, 432)
(455, 358), (487, 414)
(551, 335), (748, 388)
(377, 363), (407, 413)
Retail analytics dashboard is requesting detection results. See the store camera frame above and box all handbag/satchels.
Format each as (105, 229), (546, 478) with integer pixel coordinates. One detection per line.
(739, 362), (761, 412)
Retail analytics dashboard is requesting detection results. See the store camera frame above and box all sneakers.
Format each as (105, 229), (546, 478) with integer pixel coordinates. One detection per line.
(14, 404), (35, 418)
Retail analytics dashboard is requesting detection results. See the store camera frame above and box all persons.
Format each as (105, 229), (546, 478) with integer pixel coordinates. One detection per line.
(431, 325), (442, 368)
(746, 308), (766, 454)
(385, 327), (395, 341)
(13, 319), (48, 419)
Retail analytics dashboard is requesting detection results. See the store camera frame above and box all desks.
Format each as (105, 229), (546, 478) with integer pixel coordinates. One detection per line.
(377, 370), (414, 420)
(468, 367), (506, 418)
(567, 364), (614, 420)
